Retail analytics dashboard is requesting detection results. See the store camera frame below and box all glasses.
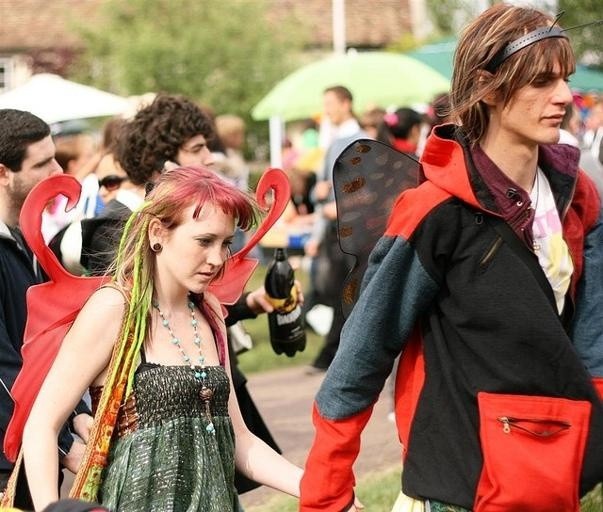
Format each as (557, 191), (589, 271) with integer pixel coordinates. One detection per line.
(98, 175), (130, 191)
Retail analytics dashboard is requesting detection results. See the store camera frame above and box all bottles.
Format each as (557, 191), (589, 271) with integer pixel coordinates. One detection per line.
(264, 248), (306, 358)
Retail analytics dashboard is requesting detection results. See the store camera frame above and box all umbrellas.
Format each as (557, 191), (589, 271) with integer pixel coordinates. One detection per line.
(0, 72), (142, 124)
(405, 37), (603, 90)
(247, 46), (454, 119)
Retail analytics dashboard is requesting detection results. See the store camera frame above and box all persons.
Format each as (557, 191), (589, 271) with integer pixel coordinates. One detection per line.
(35, 92), (283, 494)
(20, 164), (366, 512)
(52, 109), (257, 355)
(0, 107), (97, 512)
(278, 86), (603, 375)
(298, 3), (603, 512)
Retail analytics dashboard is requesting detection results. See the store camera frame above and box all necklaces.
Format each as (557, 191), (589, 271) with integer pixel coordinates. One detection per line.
(531, 167), (542, 251)
(149, 295), (216, 437)
